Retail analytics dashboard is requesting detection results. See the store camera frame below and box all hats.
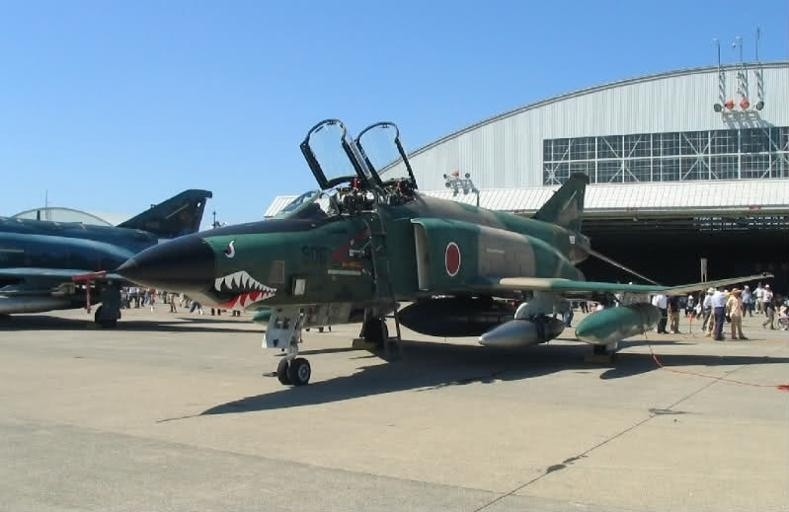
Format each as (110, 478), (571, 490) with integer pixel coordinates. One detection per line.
(744, 285), (750, 289)
(766, 284), (770, 287)
(688, 295), (694, 300)
(732, 288), (741, 293)
(707, 287), (716, 293)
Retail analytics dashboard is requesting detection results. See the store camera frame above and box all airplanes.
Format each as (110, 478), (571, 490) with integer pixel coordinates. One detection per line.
(0, 189), (213, 326)
(116, 117), (774, 386)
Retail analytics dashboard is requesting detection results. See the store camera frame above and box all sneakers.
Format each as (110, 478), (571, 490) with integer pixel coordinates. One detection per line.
(732, 335), (748, 339)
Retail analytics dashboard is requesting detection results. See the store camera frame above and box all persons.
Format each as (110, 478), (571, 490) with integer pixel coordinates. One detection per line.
(553, 299), (601, 329)
(122, 284), (241, 317)
(652, 282), (789, 342)
